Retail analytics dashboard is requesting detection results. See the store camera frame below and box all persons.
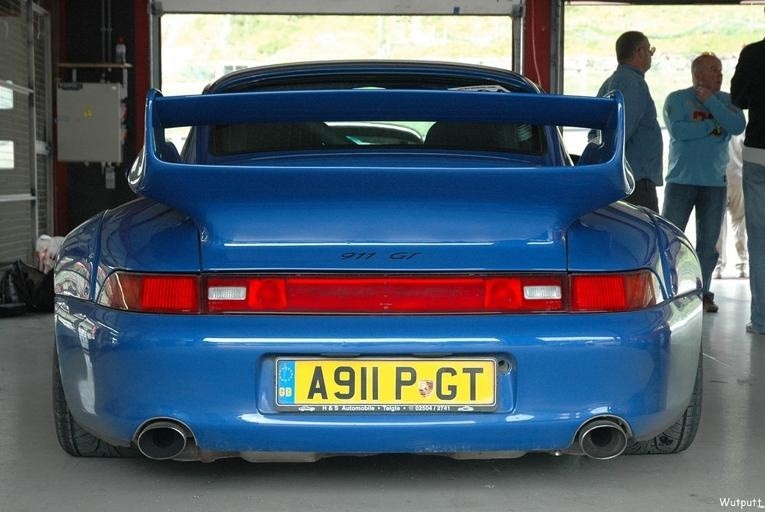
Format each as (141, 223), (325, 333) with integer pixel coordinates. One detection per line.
(661, 55), (746, 313)
(588, 31), (663, 215)
(714, 135), (749, 279)
(731, 37), (765, 334)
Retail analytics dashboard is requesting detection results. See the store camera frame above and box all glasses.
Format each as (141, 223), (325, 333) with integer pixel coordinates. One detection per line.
(647, 46), (656, 55)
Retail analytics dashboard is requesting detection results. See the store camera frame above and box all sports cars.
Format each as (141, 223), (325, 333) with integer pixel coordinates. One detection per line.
(53, 59), (705, 461)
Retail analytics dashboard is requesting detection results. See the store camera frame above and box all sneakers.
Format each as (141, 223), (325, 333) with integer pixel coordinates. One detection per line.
(713, 271), (748, 279)
(703, 291), (717, 312)
(747, 323), (765, 334)
